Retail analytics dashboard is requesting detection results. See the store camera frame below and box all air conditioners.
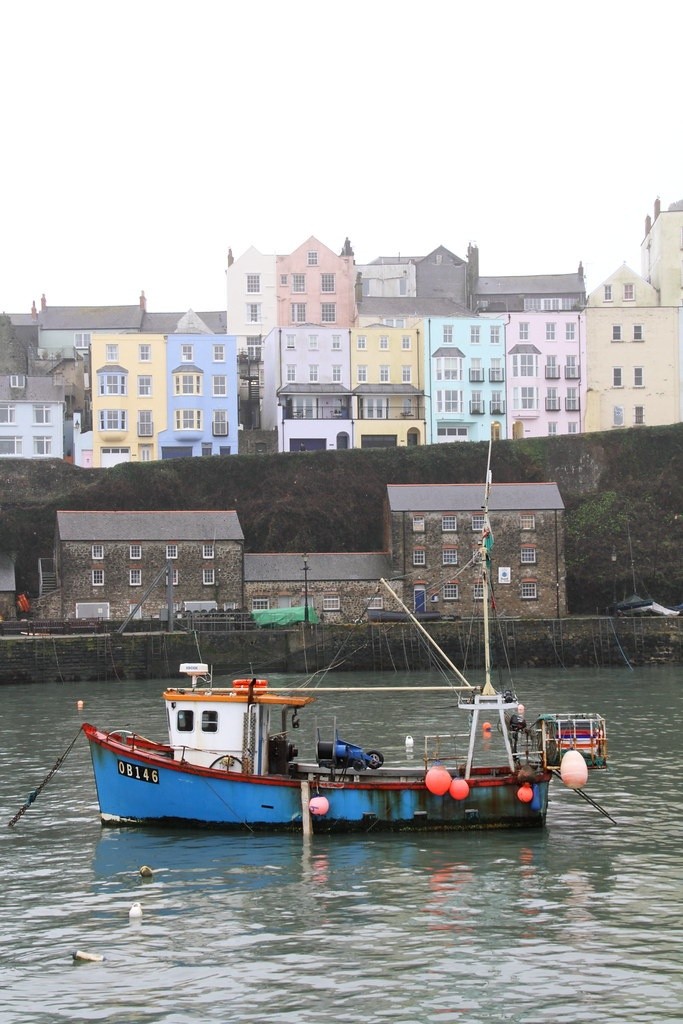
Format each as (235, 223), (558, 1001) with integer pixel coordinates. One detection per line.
(10, 374), (25, 389)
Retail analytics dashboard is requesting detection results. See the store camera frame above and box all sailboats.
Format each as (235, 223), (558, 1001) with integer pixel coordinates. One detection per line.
(610, 517), (655, 614)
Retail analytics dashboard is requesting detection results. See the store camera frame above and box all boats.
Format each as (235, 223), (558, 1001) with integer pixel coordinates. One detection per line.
(364, 607), (442, 621)
(650, 601), (680, 616)
(530, 708), (610, 771)
(80, 438), (555, 828)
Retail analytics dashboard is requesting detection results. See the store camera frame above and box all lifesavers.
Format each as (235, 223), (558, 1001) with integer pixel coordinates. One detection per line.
(17, 594), (29, 612)
(232, 679), (268, 695)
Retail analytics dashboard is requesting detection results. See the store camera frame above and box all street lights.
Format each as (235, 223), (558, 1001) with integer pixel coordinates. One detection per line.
(300, 550), (313, 626)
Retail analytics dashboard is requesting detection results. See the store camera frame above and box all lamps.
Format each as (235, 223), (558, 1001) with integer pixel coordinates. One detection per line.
(74, 420), (80, 430)
